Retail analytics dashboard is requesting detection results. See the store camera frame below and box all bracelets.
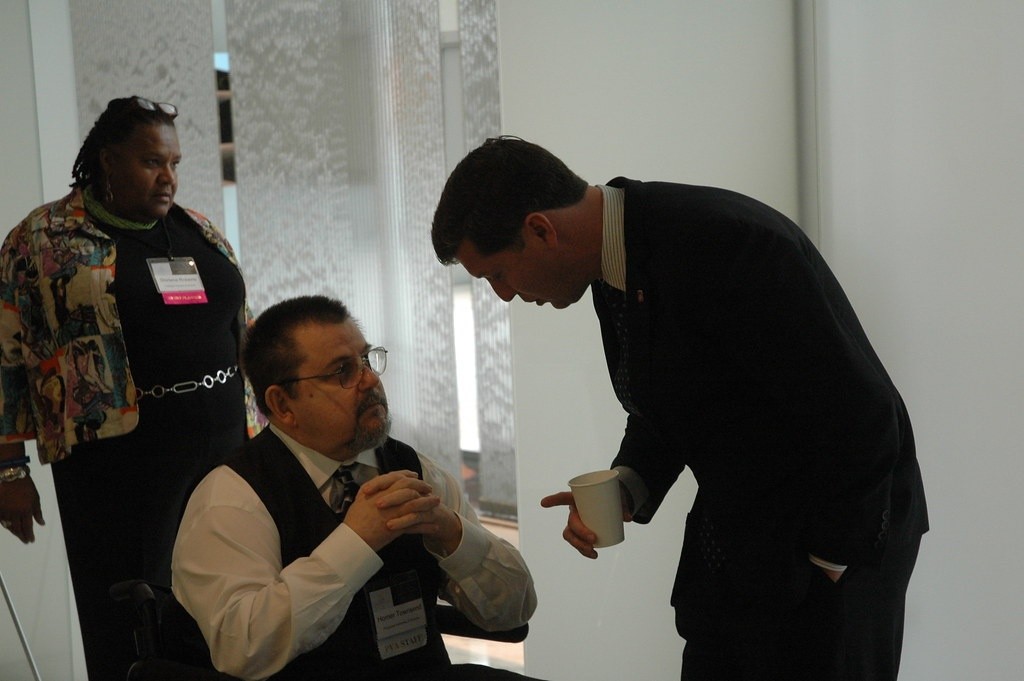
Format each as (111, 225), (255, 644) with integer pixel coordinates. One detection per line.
(0, 456), (31, 483)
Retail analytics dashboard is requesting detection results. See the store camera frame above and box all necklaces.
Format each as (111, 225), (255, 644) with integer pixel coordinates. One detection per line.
(83, 184), (158, 230)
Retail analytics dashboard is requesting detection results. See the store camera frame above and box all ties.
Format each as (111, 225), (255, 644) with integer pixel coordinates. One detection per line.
(597, 278), (725, 581)
(334, 462), (361, 513)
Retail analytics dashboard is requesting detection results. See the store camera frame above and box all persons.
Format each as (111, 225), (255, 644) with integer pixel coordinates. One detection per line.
(431, 136), (930, 681)
(0, 95), (270, 681)
(158, 295), (537, 681)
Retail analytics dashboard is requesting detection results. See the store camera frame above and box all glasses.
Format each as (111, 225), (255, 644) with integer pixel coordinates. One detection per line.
(129, 95), (178, 120)
(288, 347), (388, 389)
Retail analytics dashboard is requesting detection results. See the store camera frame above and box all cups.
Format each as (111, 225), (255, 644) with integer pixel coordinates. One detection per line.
(567, 469), (627, 549)
